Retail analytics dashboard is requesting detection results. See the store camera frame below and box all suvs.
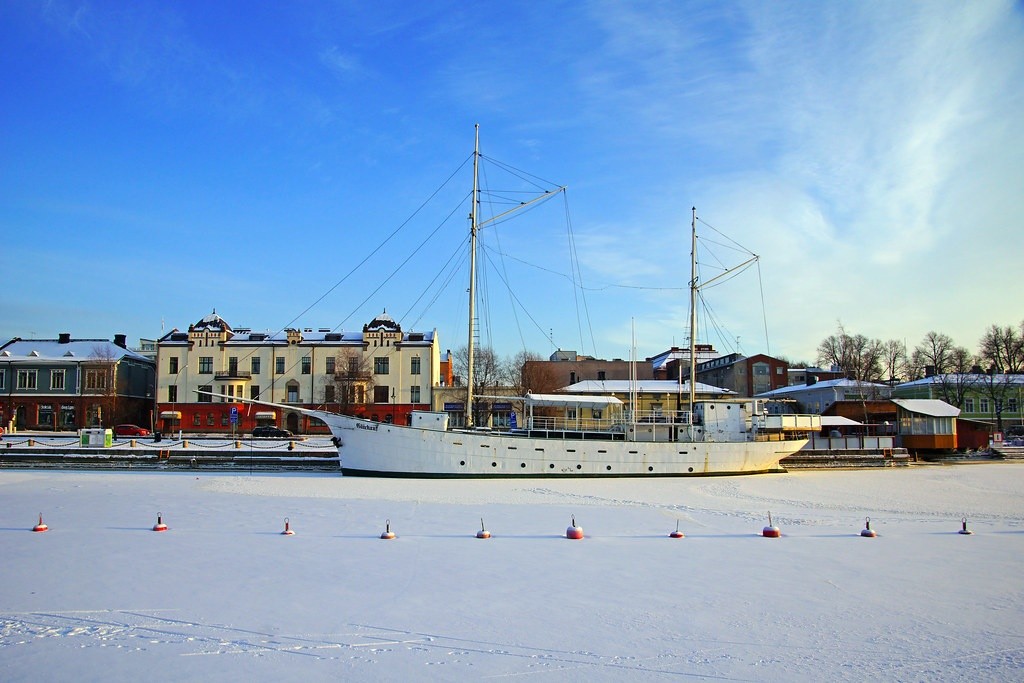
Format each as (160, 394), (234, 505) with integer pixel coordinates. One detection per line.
(115, 424), (149, 436)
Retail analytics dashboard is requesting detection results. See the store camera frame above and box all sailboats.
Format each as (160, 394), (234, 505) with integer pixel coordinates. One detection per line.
(192, 123), (812, 478)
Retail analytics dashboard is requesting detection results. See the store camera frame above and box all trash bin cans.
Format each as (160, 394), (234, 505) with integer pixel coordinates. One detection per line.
(80, 428), (113, 448)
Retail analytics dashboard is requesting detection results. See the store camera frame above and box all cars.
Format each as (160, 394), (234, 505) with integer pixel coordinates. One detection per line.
(253, 426), (291, 437)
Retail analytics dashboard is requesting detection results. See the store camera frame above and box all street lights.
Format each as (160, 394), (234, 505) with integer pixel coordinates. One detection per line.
(172, 364), (188, 436)
